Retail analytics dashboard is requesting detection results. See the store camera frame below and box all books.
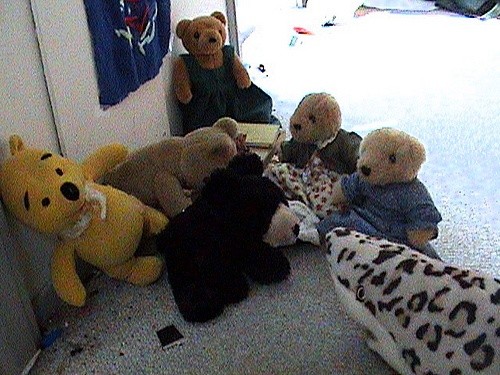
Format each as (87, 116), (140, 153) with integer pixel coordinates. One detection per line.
(236, 122), (286, 165)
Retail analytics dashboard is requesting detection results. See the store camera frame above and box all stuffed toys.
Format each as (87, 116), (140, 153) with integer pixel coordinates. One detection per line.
(1, 135), (170, 307)
(316, 127), (438, 259)
(269, 93), (362, 216)
(157, 150), (300, 324)
(325, 227), (500, 375)
(175, 11), (272, 128)
(105, 117), (239, 214)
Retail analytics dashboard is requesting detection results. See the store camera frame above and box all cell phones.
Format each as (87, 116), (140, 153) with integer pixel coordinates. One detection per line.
(156, 323), (186, 350)
